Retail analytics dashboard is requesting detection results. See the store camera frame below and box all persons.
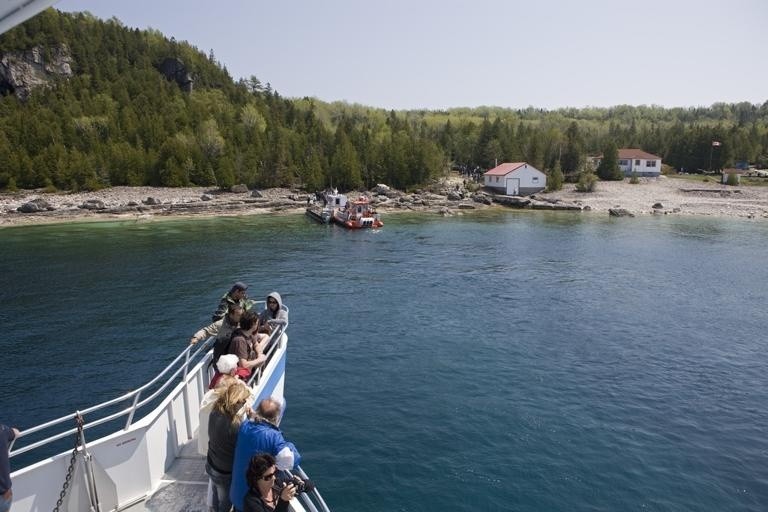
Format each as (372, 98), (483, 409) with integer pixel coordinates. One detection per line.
(305, 196), (311, 203)
(242, 452), (317, 512)
(208, 353), (251, 390)
(226, 308), (268, 371)
(193, 373), (258, 512)
(256, 290), (289, 349)
(455, 160), (482, 191)
(189, 304), (245, 362)
(202, 378), (257, 512)
(228, 396), (303, 512)
(211, 281), (257, 323)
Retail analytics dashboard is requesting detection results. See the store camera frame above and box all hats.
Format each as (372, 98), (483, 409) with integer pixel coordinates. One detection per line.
(216, 354), (239, 375)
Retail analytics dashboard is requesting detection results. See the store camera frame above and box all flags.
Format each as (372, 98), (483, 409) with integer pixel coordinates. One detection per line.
(710, 137), (722, 148)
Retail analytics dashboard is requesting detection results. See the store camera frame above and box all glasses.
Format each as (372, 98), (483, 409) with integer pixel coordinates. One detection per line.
(268, 300), (277, 304)
(260, 472), (276, 481)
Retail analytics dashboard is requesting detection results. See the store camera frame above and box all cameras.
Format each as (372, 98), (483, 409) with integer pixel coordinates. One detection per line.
(282, 476), (306, 493)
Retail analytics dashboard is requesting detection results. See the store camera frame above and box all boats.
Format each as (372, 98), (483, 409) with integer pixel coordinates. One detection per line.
(0, 301), (331, 512)
(306, 187), (384, 229)
